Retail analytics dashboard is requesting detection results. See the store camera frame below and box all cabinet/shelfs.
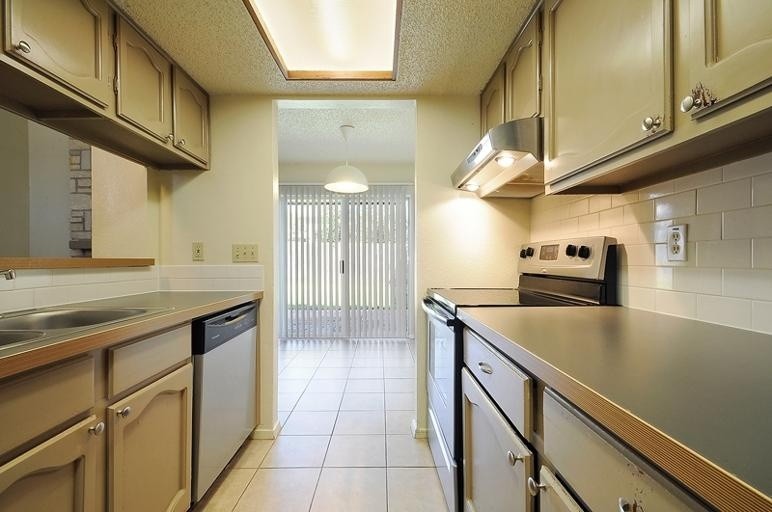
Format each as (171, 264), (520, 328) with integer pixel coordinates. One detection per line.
(0, 324), (193, 511)
(114, 18), (210, 171)
(457, 326), (714, 511)
(0, 0), (115, 125)
(480, 12), (542, 139)
(543, 0), (772, 194)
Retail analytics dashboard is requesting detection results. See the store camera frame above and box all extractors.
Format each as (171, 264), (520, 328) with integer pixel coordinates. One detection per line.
(450, 116), (543, 198)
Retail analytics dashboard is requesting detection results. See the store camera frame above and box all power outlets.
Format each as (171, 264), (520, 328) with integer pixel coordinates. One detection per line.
(667, 224), (687, 261)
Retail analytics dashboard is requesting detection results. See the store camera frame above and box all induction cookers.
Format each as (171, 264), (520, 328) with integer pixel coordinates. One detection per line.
(426, 275), (606, 315)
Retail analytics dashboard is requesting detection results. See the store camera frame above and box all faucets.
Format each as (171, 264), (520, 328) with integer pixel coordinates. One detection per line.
(0, 266), (16, 281)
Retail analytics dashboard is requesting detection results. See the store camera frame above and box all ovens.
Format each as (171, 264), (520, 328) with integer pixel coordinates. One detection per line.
(425, 298), (465, 512)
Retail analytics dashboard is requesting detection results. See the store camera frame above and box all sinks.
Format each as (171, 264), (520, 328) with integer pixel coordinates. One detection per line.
(0, 305), (175, 330)
(0, 330), (47, 350)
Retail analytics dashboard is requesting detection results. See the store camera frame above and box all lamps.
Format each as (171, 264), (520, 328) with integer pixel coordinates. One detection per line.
(324, 125), (369, 194)
